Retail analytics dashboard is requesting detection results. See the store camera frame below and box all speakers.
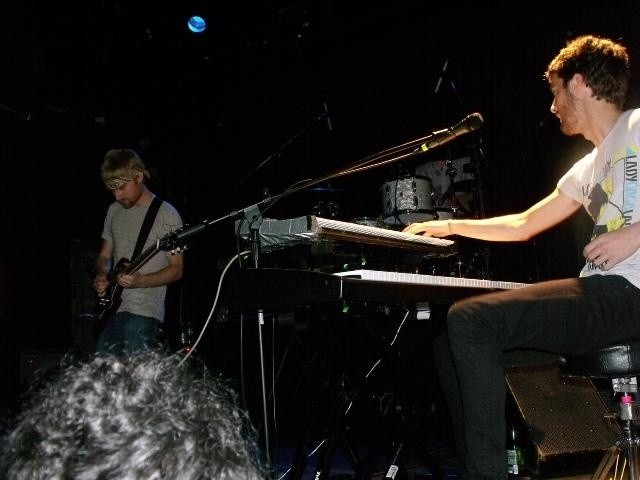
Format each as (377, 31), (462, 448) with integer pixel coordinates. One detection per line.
(505, 361), (640, 480)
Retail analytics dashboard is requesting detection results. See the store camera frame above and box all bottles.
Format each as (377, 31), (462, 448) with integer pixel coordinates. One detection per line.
(507, 414), (530, 478)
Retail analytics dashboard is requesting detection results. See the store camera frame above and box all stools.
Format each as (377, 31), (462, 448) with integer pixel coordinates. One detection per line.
(560, 347), (638, 480)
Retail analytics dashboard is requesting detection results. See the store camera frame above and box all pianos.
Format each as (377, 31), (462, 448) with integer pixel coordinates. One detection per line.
(240, 269), (536, 299)
(236, 216), (463, 256)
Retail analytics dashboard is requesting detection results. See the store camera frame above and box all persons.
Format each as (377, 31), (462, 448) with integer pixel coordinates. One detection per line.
(401, 36), (640, 479)
(93, 149), (188, 357)
(1, 343), (268, 480)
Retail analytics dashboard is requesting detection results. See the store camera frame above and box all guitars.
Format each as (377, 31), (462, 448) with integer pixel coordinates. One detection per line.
(96, 224), (194, 332)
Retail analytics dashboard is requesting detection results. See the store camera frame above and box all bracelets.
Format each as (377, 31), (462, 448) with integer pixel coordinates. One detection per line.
(448, 219), (452, 233)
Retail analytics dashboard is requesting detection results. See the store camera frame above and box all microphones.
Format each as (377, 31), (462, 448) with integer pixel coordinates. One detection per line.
(323, 102), (333, 131)
(413, 112), (485, 154)
(434, 59), (449, 94)
